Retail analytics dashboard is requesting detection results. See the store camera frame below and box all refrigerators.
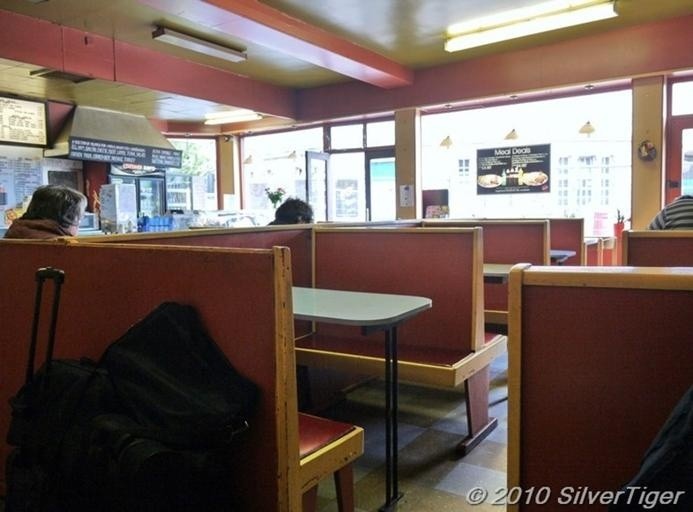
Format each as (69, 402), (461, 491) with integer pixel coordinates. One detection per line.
(84, 162), (167, 218)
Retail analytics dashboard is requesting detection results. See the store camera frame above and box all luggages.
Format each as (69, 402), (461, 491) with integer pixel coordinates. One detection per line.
(4, 267), (66, 511)
(68, 411), (223, 511)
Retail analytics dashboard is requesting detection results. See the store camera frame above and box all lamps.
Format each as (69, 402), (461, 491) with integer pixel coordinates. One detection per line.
(443, 0), (624, 53)
(152, 28), (246, 64)
(205, 114), (262, 125)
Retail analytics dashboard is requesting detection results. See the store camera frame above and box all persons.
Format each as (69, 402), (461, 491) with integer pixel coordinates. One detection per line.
(646, 194), (693, 233)
(4, 184), (89, 239)
(268, 198), (313, 226)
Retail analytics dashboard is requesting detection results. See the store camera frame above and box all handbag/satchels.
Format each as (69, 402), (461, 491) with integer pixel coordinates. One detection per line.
(5, 353), (114, 449)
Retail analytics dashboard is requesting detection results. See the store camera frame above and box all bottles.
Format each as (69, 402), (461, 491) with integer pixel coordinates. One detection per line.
(505, 159), (524, 186)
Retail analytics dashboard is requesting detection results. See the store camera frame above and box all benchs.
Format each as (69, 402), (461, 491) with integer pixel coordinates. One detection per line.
(0, 215), (693, 512)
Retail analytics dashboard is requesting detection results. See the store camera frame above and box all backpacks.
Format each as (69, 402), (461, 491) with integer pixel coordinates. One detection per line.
(93, 300), (257, 450)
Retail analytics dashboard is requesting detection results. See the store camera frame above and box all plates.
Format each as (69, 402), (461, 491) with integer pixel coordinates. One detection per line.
(477, 174), (503, 188)
(522, 172), (548, 186)
